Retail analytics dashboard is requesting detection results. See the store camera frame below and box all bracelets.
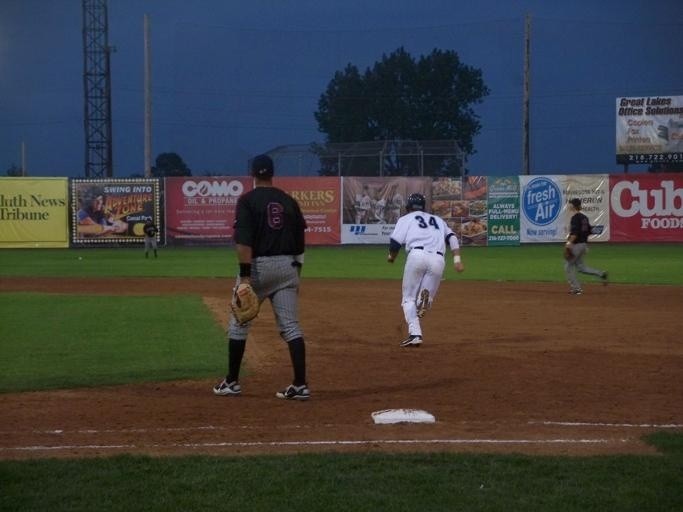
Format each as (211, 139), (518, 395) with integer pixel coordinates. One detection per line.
(239, 262), (252, 277)
(453, 255), (461, 263)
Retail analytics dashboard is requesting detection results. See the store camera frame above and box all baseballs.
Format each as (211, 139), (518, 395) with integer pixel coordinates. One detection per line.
(78, 257), (83, 261)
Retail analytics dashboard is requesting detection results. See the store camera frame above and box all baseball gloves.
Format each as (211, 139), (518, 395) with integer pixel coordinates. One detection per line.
(230, 283), (259, 324)
(564, 247), (575, 260)
(148, 230), (155, 237)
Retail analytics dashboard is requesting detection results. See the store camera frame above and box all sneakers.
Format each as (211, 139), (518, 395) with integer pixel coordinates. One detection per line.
(213, 379), (241, 395)
(602, 271), (608, 286)
(568, 288), (583, 295)
(400, 335), (423, 347)
(417, 288), (429, 318)
(276, 384), (310, 401)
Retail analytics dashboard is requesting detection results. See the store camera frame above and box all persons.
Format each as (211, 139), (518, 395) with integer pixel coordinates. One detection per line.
(112, 219), (146, 236)
(76, 187), (113, 234)
(564, 198), (608, 295)
(387, 192), (464, 346)
(353, 184), (404, 224)
(212, 154), (311, 400)
(144, 218), (158, 258)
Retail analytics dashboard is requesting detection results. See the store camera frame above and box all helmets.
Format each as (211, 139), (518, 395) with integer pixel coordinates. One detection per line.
(405, 193), (426, 209)
